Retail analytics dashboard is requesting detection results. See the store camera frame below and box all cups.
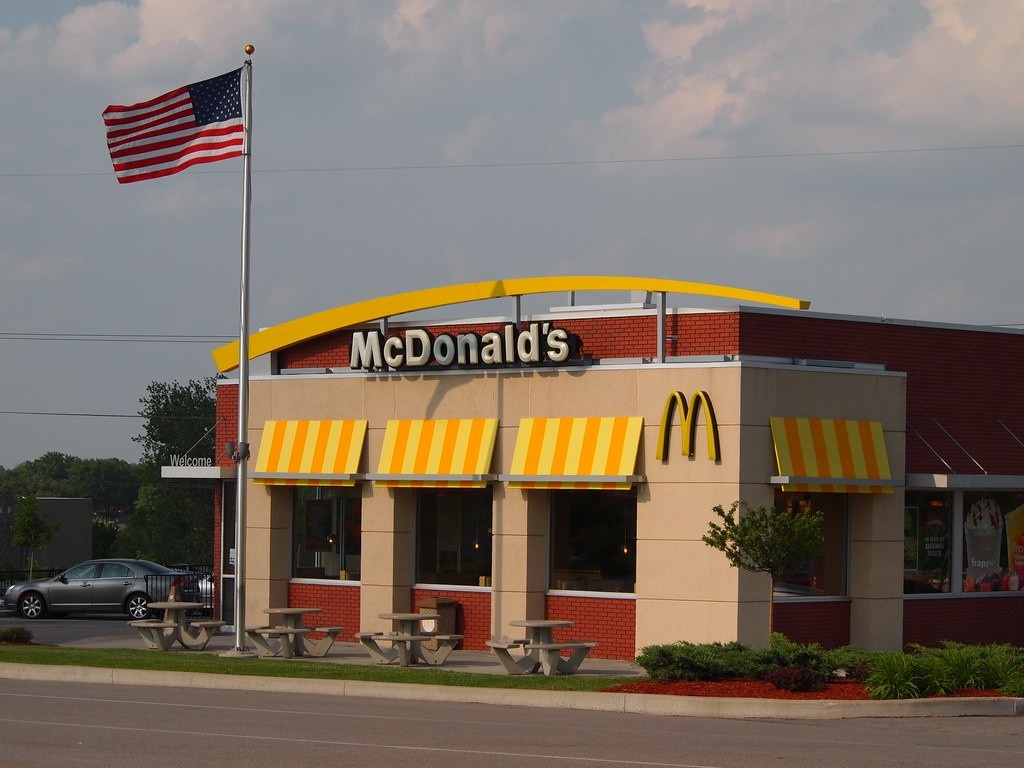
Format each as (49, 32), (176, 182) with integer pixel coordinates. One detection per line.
(979, 583), (992, 591)
(965, 525), (1002, 579)
(1003, 516), (1024, 574)
(964, 576), (976, 591)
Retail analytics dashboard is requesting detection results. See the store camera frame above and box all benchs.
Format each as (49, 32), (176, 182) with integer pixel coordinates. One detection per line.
(127, 618), (226, 651)
(486, 639), (599, 676)
(245, 624), (347, 658)
(354, 631), (463, 667)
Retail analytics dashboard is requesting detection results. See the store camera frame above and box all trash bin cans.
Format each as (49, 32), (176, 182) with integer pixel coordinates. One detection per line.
(416, 596), (459, 649)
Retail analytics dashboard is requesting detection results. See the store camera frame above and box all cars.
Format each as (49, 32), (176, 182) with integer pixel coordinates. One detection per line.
(2, 558), (203, 620)
(197, 571), (215, 599)
(903, 574), (937, 594)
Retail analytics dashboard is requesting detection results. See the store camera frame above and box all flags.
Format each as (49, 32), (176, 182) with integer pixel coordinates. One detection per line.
(101, 66), (251, 183)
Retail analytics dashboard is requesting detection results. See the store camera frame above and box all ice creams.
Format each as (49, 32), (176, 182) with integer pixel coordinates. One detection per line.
(964, 496), (1003, 579)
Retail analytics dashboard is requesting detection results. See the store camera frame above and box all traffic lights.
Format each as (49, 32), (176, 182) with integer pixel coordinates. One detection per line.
(472, 543), (480, 552)
(326, 535), (337, 545)
(624, 545), (628, 555)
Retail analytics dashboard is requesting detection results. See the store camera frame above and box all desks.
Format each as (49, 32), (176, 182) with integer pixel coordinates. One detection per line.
(509, 619), (574, 671)
(378, 613), (441, 667)
(147, 602), (205, 647)
(262, 609), (323, 656)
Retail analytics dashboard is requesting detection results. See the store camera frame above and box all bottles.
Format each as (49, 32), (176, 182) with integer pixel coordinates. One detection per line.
(1008, 572), (1019, 591)
(1002, 570), (1011, 590)
(922, 501), (947, 574)
